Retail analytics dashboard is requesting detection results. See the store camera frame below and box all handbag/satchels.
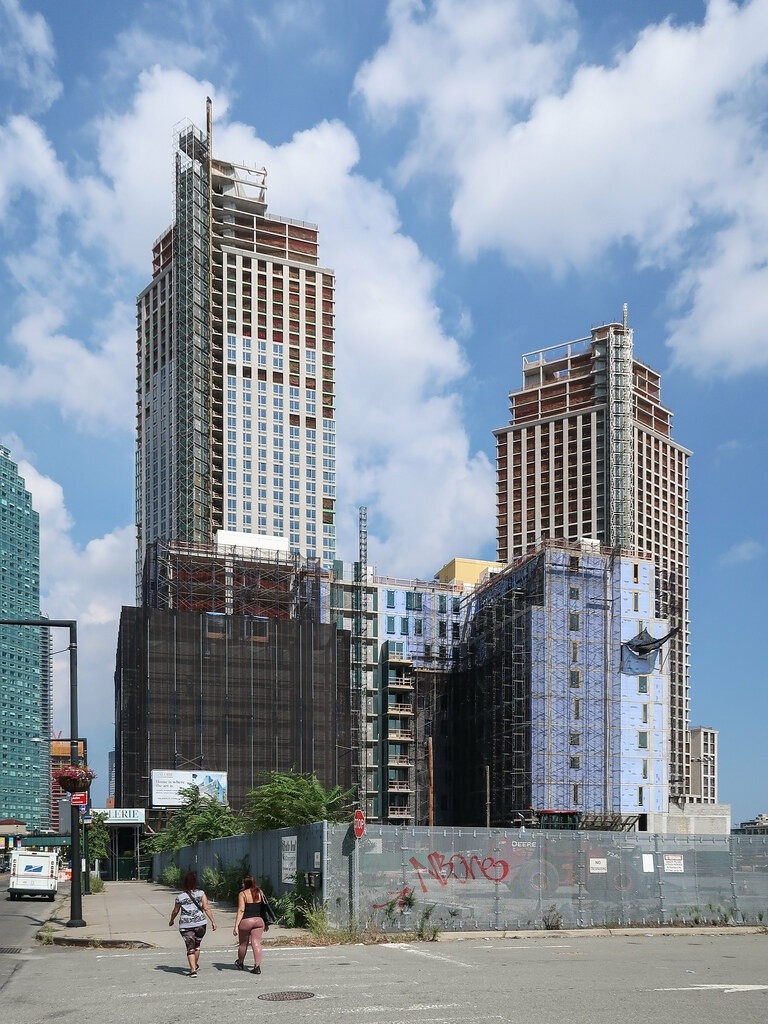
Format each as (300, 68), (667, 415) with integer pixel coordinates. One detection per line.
(261, 889), (276, 926)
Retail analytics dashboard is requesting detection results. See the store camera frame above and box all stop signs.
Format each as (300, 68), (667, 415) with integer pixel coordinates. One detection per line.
(354, 810), (366, 840)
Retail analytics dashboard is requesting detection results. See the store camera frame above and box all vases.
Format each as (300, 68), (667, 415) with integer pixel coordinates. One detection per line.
(56, 777), (92, 792)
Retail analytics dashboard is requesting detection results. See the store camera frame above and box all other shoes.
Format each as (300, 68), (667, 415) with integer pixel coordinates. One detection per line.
(186, 971), (198, 978)
(250, 965), (261, 974)
(235, 958), (243, 970)
(195, 964), (199, 970)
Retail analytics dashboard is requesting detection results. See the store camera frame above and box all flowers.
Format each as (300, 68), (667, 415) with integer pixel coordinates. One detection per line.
(52, 764), (97, 781)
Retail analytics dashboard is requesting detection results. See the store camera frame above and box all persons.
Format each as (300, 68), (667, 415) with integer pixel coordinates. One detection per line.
(233, 876), (269, 974)
(168, 872), (216, 978)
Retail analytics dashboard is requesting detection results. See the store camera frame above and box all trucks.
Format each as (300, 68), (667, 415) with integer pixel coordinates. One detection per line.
(7, 850), (63, 902)
